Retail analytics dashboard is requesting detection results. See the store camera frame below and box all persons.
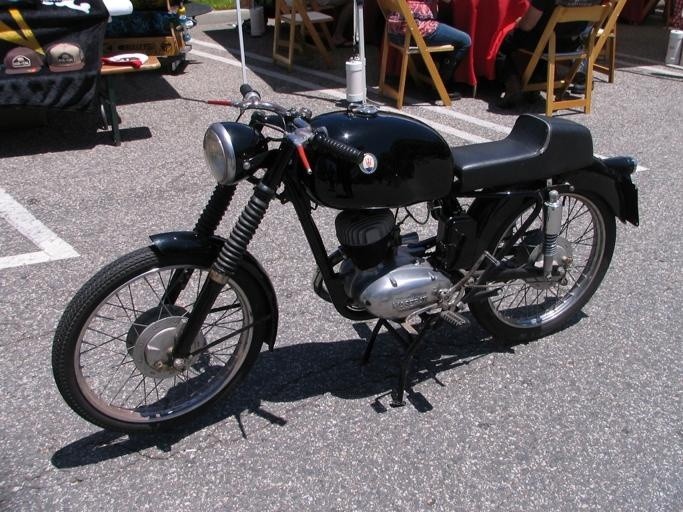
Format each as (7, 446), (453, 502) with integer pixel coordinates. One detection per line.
(488, 0), (604, 111)
(387, 0), (473, 100)
(284, 0), (355, 48)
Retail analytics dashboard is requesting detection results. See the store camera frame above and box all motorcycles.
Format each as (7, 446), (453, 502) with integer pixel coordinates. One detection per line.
(49, 83), (641, 436)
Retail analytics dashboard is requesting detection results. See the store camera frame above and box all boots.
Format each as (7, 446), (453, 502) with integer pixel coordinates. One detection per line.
(494, 75), (522, 109)
(429, 54), (462, 101)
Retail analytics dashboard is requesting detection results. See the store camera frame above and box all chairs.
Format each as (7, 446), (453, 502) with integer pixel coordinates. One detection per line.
(270, 0), (335, 74)
(376, 1), (454, 111)
(515, 0), (627, 117)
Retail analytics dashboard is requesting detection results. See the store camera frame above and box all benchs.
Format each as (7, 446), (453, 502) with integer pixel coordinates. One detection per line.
(0, 54), (161, 145)
